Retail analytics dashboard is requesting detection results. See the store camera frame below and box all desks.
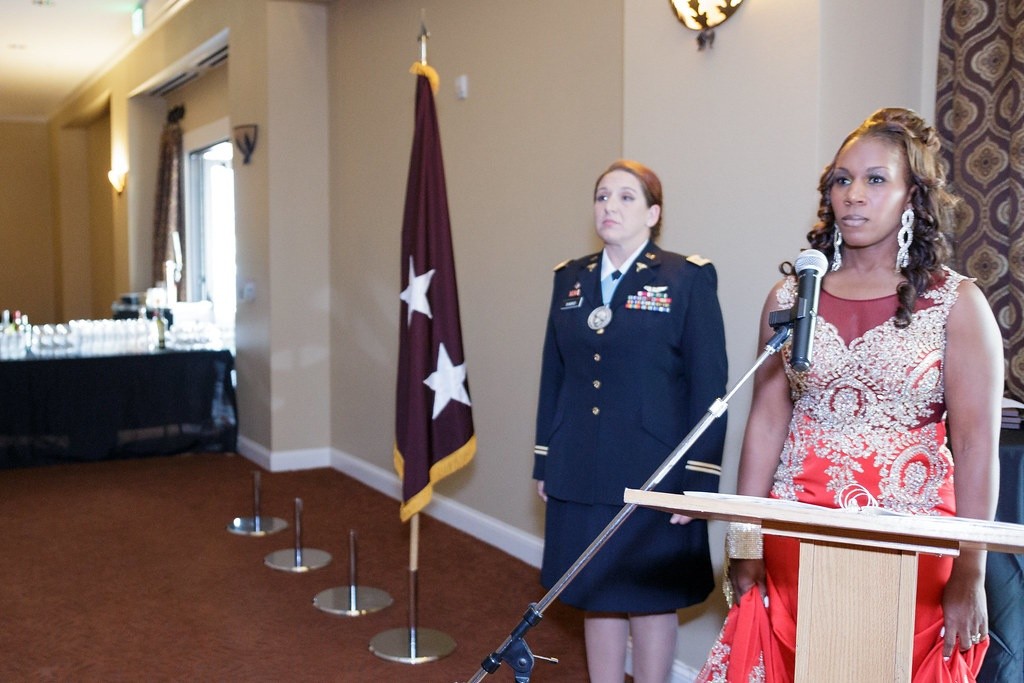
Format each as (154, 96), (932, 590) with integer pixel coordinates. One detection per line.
(2, 348), (245, 462)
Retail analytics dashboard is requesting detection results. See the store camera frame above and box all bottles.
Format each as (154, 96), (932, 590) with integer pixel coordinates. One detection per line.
(0, 309), (168, 361)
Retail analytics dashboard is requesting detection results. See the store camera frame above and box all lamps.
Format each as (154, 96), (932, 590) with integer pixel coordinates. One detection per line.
(231, 125), (256, 163)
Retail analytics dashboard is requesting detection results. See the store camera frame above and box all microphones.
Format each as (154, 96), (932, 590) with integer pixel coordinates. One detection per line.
(789, 249), (828, 372)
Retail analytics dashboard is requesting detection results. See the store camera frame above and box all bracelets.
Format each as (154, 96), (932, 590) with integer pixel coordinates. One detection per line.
(729, 520), (764, 560)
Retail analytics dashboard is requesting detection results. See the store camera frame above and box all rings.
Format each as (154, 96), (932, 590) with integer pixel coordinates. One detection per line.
(972, 633), (982, 642)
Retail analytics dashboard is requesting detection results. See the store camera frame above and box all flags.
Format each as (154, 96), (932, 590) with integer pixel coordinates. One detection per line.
(393, 61), (475, 521)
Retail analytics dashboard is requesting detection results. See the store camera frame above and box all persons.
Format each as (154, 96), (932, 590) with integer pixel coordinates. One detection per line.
(532, 159), (729, 683)
(696, 107), (1006, 683)
